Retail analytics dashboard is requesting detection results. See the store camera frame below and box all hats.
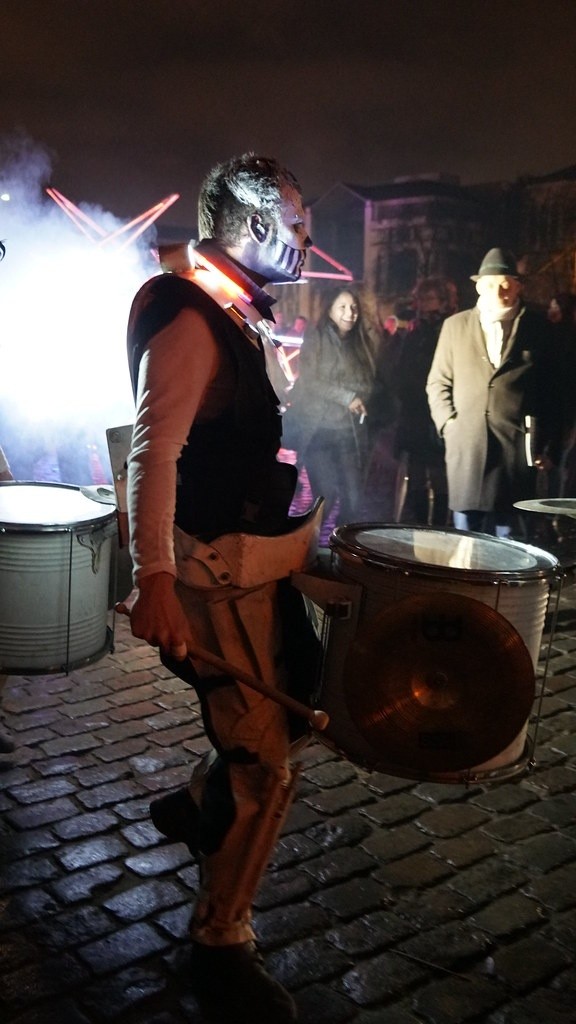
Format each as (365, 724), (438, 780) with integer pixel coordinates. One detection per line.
(469, 247), (525, 284)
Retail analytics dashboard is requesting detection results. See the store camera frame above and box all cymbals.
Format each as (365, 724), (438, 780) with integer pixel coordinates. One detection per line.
(513, 497), (575, 516)
(341, 591), (536, 773)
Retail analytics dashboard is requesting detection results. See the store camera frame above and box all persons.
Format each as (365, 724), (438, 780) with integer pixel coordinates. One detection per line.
(127, 153), (317, 1024)
(400, 279), (459, 525)
(293, 287), (377, 525)
(427, 246), (576, 536)
(272, 295), (576, 371)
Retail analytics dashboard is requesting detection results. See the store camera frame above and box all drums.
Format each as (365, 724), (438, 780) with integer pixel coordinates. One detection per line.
(0, 479), (121, 677)
(313, 520), (564, 789)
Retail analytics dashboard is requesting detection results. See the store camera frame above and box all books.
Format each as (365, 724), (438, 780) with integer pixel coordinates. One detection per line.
(525, 414), (552, 467)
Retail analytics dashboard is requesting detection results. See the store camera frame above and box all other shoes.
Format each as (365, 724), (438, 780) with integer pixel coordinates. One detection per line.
(173, 944), (296, 1023)
(151, 789), (206, 867)
